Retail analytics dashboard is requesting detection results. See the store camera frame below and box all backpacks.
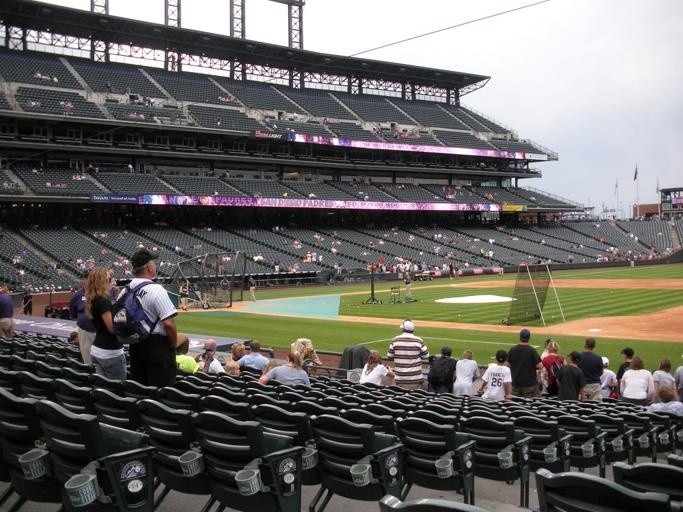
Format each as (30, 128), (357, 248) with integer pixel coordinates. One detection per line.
(110, 282), (160, 344)
(428, 359), (451, 384)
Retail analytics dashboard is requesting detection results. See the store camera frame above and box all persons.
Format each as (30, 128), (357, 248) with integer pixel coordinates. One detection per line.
(115, 249), (176, 388)
(68, 332), (78, 346)
(1, 65), (683, 311)
(84, 267), (127, 383)
(359, 318), (682, 418)
(20, 282), (33, 316)
(0, 286), (13, 338)
(174, 332), (322, 389)
(70, 273), (96, 368)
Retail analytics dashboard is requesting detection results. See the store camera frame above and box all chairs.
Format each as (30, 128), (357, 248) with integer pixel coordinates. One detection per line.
(1, 330), (682, 511)
(0, 47), (682, 297)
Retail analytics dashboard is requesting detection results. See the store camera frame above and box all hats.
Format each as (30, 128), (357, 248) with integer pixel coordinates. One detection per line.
(621, 348), (633, 355)
(131, 248), (159, 267)
(602, 356), (610, 366)
(520, 330), (530, 338)
(497, 351), (506, 362)
(400, 319), (414, 332)
(567, 350), (581, 363)
